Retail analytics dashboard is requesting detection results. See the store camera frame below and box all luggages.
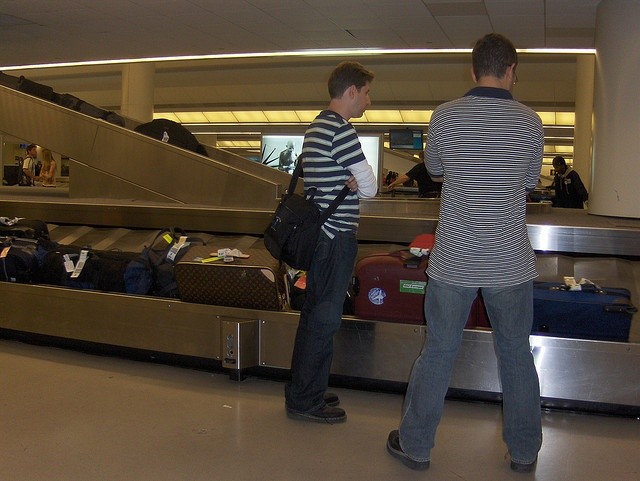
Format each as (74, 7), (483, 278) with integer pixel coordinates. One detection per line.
(532, 277), (638, 341)
(352, 249), (479, 329)
(173, 246), (290, 311)
(1, 237), (39, 284)
(1, 217), (51, 241)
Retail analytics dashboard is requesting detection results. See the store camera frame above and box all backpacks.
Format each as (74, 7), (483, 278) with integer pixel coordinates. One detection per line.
(126, 227), (206, 300)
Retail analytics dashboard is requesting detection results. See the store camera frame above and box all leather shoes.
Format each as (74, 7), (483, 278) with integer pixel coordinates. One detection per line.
(285, 393), (340, 409)
(287, 400), (347, 423)
(511, 460), (536, 474)
(387, 430), (430, 470)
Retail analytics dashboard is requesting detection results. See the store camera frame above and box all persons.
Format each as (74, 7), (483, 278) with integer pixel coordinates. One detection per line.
(284, 61), (378, 425)
(278, 140), (298, 172)
(40, 148), (57, 187)
(21, 144), (39, 187)
(387, 151), (442, 198)
(542, 154), (587, 209)
(385, 32), (545, 473)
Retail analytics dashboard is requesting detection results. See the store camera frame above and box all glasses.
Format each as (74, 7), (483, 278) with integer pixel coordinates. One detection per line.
(513, 73), (518, 85)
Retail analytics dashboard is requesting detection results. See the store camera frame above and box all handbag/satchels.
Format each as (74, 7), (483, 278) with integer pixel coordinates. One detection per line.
(264, 153), (350, 271)
(42, 245), (124, 296)
